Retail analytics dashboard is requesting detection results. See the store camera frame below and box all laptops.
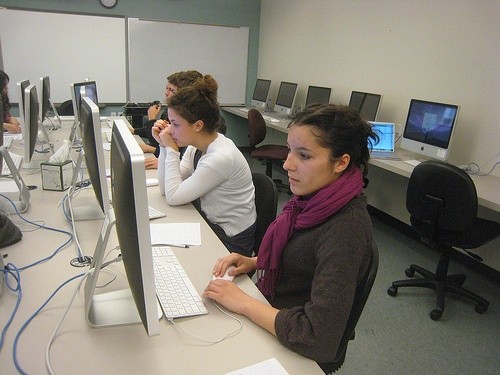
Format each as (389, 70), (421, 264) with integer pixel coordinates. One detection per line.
(364, 121), (396, 160)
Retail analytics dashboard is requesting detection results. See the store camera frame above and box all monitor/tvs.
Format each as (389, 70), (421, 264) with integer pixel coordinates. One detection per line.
(20, 85), (54, 163)
(40, 76), (62, 131)
(15, 80), (48, 144)
(305, 86), (332, 106)
(348, 91), (383, 122)
(85, 119), (164, 336)
(274, 81), (299, 115)
(401, 98), (461, 162)
(251, 78), (272, 113)
(66, 83), (99, 144)
(67, 96), (109, 220)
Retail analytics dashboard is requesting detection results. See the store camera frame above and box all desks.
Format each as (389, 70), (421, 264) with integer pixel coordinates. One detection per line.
(0, 116), (325, 375)
(221, 106), (500, 214)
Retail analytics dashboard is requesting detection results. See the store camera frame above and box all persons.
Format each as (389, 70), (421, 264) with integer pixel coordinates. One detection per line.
(147, 70), (257, 258)
(1, 72), (22, 133)
(202, 102), (383, 375)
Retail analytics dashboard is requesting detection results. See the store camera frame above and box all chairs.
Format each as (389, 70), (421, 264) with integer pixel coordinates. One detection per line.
(388, 161), (500, 321)
(247, 109), (293, 196)
(250, 173), (279, 279)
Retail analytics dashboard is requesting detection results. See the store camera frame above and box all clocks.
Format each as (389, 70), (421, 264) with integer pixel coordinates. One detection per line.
(99, 0), (118, 9)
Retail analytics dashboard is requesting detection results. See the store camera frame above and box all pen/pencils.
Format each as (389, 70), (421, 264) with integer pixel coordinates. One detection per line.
(169, 244), (189, 248)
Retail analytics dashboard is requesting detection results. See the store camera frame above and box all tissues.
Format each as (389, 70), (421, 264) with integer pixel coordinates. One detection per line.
(39, 139), (74, 192)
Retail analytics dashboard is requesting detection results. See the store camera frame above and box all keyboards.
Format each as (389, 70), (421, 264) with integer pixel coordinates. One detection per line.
(261, 114), (280, 123)
(151, 247), (208, 319)
(2, 136), (13, 148)
(405, 159), (421, 167)
(106, 119), (113, 128)
(238, 108), (250, 112)
(106, 132), (112, 142)
(147, 207), (166, 220)
(1, 152), (22, 175)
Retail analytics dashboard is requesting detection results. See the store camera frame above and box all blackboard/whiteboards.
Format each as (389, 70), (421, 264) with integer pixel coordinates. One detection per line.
(0, 7), (126, 107)
(126, 5), (250, 107)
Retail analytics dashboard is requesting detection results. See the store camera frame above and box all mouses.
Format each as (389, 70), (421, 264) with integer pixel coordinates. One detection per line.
(211, 265), (236, 282)
